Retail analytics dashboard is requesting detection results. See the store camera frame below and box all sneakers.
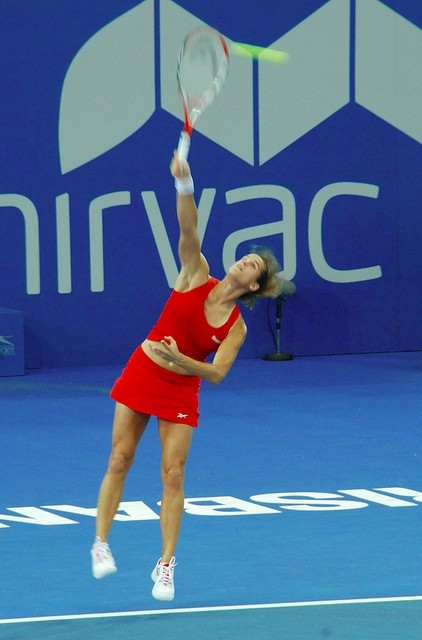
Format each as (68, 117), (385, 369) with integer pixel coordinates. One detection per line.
(91, 537), (117, 580)
(151, 557), (179, 602)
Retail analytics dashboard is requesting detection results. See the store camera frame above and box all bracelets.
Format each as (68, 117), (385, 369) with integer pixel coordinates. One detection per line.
(173, 178), (194, 195)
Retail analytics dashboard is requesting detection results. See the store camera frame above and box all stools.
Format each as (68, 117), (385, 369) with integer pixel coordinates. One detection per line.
(259, 280), (297, 360)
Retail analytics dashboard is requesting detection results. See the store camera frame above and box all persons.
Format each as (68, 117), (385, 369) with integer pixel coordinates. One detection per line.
(92, 148), (280, 601)
